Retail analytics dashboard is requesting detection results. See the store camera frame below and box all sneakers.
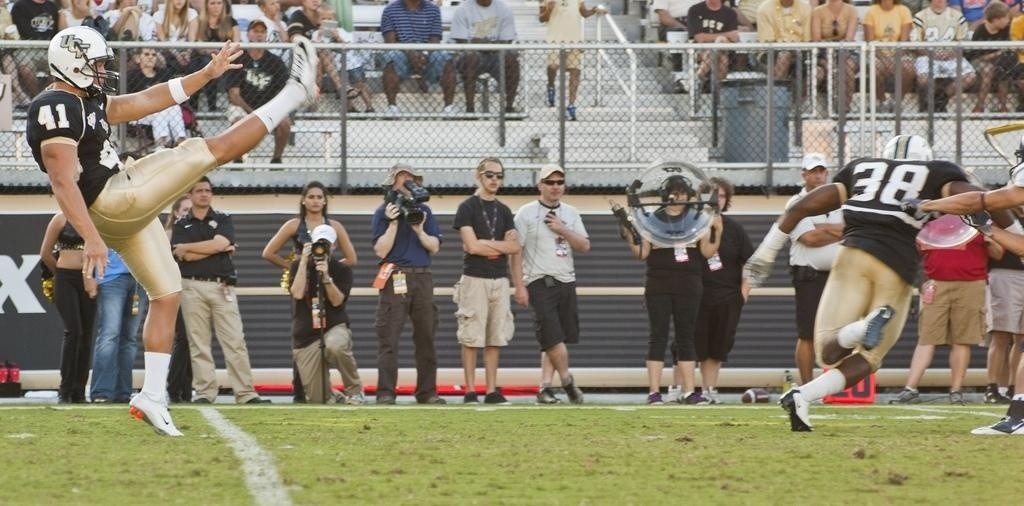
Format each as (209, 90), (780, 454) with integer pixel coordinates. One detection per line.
(1005, 384), (1014, 401)
(777, 388), (815, 431)
(950, 390), (963, 404)
(285, 35), (319, 108)
(331, 387), (447, 404)
(970, 414), (1024, 436)
(982, 389), (997, 403)
(888, 387), (920, 405)
(463, 374), (584, 405)
(129, 387), (185, 436)
(346, 85), (456, 119)
(647, 384), (724, 406)
(860, 304), (895, 350)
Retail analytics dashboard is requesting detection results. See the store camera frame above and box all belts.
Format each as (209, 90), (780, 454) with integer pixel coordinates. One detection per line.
(398, 266), (432, 273)
(182, 274), (226, 282)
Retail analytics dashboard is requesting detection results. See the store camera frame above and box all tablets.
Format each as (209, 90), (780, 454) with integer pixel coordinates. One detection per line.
(322, 21), (337, 38)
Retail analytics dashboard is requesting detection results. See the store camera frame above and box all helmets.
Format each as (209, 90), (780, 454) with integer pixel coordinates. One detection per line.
(48, 24), (121, 97)
(1013, 134), (1024, 158)
(883, 132), (933, 160)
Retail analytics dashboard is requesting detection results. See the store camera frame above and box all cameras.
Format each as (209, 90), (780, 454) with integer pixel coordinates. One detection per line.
(311, 241), (329, 261)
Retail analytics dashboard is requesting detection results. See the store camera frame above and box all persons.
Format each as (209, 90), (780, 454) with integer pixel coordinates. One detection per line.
(645, 0), (1023, 109)
(741, 137), (1024, 436)
(773, 154), (1022, 405)
(28, 24), (321, 441)
(897, 158), (1024, 436)
(0, 1), (607, 173)
(41, 194), (142, 406)
(167, 157), (757, 406)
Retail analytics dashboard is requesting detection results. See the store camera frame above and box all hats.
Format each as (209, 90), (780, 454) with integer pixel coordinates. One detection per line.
(312, 224), (338, 244)
(248, 19), (268, 29)
(539, 163), (565, 180)
(801, 151), (827, 170)
(381, 162), (423, 191)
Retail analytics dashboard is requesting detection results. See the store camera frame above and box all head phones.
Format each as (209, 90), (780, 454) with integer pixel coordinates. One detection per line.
(658, 175), (695, 199)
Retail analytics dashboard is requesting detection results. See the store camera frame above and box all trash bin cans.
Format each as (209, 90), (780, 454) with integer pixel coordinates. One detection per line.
(717, 77), (791, 166)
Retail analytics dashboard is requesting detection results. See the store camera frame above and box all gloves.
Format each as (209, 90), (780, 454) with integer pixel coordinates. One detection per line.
(901, 197), (931, 221)
(959, 209), (993, 229)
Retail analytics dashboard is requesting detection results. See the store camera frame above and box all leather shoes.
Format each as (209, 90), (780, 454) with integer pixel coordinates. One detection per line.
(246, 396), (272, 403)
(192, 397), (211, 403)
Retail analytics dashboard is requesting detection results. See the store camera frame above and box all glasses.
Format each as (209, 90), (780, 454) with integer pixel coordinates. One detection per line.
(479, 169), (504, 178)
(543, 178), (565, 185)
